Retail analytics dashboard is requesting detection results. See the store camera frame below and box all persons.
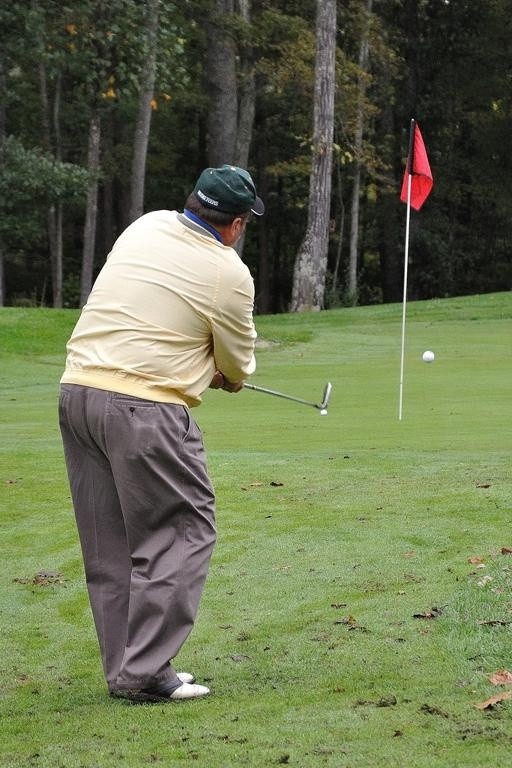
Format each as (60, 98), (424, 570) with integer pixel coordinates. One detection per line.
(53, 165), (267, 705)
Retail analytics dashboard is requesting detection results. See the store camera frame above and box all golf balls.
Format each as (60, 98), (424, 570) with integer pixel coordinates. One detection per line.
(321, 410), (327, 415)
(423, 351), (434, 362)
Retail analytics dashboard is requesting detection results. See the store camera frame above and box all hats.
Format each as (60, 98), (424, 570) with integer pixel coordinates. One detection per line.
(194, 163), (265, 216)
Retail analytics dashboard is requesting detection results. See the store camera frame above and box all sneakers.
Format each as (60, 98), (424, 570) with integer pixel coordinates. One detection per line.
(110, 672), (211, 702)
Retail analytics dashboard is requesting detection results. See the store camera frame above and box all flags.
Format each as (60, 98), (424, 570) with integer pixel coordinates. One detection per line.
(399, 119), (437, 214)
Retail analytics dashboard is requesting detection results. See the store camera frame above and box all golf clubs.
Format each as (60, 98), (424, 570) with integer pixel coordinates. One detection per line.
(244, 382), (332, 408)
